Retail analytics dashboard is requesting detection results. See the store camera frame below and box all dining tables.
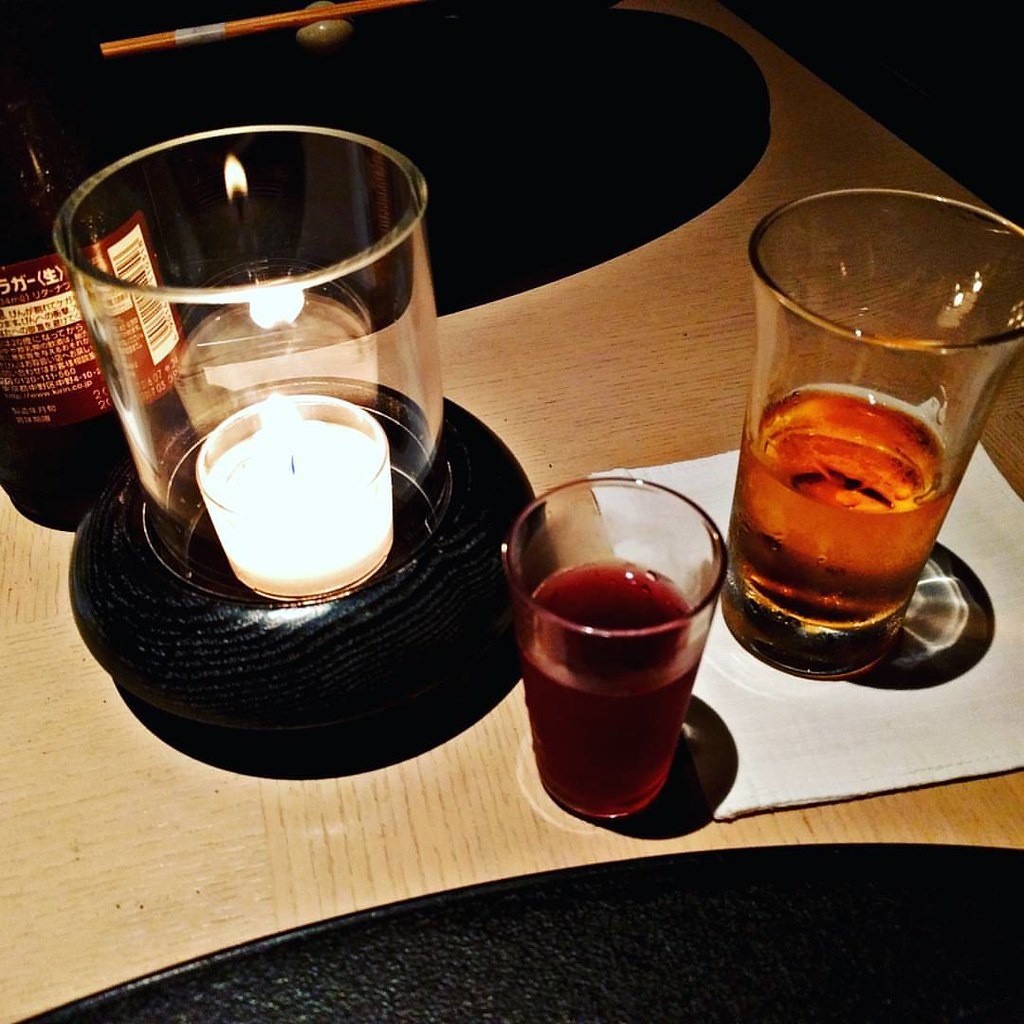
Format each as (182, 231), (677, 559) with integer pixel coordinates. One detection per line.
(0, 1), (1022, 1022)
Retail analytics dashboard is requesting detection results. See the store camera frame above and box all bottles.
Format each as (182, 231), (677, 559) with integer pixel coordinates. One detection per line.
(1, 0), (213, 532)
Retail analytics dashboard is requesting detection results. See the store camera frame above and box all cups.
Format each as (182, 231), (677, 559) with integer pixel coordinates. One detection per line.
(51, 122), (456, 610)
(501, 473), (727, 821)
(722, 185), (1024, 679)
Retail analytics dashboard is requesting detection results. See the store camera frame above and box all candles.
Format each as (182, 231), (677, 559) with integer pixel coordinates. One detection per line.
(193, 391), (396, 600)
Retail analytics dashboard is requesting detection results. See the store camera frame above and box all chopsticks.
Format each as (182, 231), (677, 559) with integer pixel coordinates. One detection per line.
(101, 0), (416, 55)
